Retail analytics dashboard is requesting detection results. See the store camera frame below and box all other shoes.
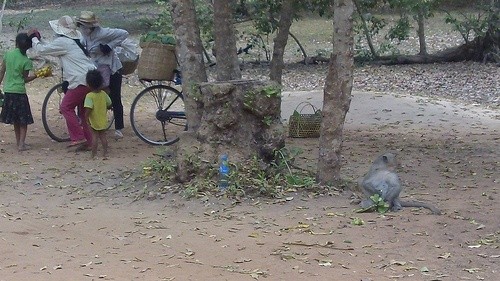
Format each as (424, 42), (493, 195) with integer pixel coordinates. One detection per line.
(74, 145), (90, 152)
(114, 130), (123, 138)
(65, 140), (87, 150)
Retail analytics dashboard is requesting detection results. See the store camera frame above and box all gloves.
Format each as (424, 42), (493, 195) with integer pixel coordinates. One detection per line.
(100, 43), (111, 55)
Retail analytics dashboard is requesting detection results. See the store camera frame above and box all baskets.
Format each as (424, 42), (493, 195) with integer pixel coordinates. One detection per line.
(119, 54), (139, 75)
(289, 101), (324, 137)
(137, 40), (176, 81)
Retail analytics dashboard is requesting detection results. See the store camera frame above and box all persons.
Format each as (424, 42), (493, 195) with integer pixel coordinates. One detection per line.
(77, 11), (129, 139)
(0, 33), (38, 151)
(27, 15), (94, 152)
(83, 70), (112, 161)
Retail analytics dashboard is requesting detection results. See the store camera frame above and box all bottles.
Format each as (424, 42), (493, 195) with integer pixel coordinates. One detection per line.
(218, 157), (229, 192)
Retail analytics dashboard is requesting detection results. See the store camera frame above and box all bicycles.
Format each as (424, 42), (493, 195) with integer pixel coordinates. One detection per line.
(42, 68), (115, 142)
(130, 45), (253, 145)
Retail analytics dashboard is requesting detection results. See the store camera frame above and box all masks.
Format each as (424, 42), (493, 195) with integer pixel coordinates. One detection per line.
(79, 26), (93, 35)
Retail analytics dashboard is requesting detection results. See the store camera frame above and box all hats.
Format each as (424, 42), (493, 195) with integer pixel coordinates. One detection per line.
(74, 10), (98, 23)
(49, 15), (82, 39)
(76, 21), (98, 28)
